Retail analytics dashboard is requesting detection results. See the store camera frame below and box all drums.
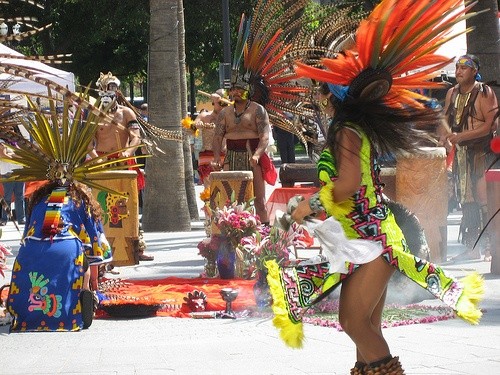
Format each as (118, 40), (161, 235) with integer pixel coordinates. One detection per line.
(80, 169), (141, 267)
(395, 145), (450, 266)
(483, 168), (500, 275)
(208, 169), (257, 238)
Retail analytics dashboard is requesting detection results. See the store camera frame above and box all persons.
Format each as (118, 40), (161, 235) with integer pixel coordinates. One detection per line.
(273, 112), (296, 164)
(181, 86), (234, 237)
(197, 79), (277, 278)
(0, 125), (27, 226)
(86, 71), (154, 261)
(265, 76), (486, 375)
(436, 55), (500, 261)
(134, 103), (148, 168)
(6, 163), (113, 335)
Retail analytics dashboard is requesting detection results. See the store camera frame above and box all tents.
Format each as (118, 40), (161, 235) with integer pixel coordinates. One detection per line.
(0, 43), (75, 123)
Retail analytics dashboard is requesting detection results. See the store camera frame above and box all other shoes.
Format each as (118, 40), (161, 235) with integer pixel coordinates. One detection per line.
(140, 251), (154, 260)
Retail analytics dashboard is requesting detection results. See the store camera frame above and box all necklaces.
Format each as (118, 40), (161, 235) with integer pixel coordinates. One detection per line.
(234, 100), (250, 118)
(107, 100), (119, 112)
